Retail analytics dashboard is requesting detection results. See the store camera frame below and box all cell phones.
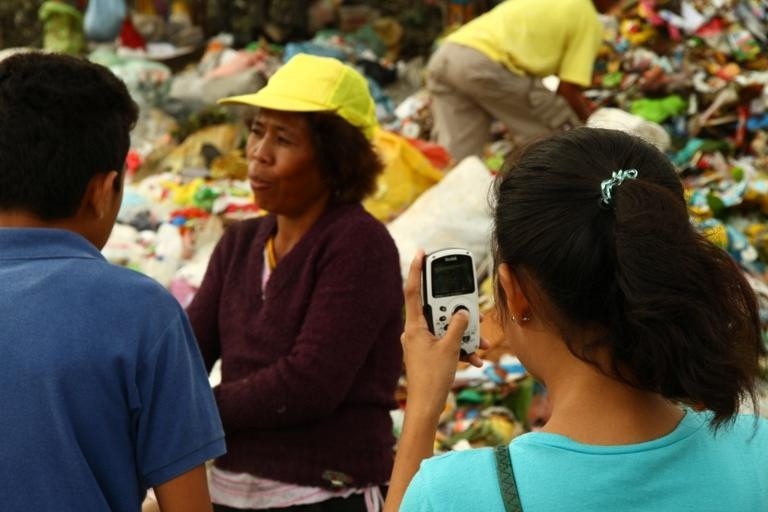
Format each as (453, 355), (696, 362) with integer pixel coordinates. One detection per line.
(422, 247), (481, 359)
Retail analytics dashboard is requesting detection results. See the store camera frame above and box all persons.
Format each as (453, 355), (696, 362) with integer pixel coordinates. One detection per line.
(380, 126), (768, 511)
(0, 50), (229, 511)
(423, 1), (628, 160)
(182, 53), (407, 511)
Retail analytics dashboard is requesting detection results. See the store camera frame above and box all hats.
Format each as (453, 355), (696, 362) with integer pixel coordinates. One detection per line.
(215, 52), (378, 148)
